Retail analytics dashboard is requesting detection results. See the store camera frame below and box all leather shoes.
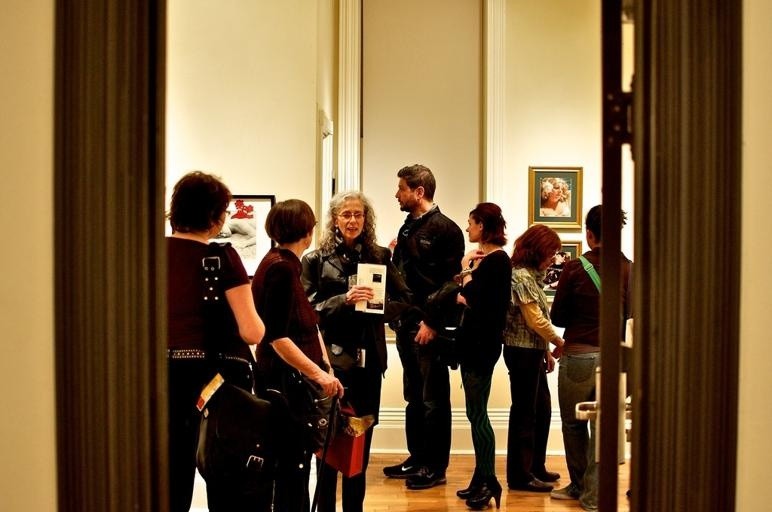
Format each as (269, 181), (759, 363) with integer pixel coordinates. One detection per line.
(537, 470), (560, 482)
(507, 478), (554, 492)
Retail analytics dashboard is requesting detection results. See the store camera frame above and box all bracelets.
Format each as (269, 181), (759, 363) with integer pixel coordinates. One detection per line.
(461, 267), (472, 279)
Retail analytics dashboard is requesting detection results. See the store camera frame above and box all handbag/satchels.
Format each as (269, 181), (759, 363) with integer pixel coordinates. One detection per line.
(195, 380), (286, 491)
(276, 368), (333, 452)
(450, 329), (459, 369)
(315, 404), (374, 478)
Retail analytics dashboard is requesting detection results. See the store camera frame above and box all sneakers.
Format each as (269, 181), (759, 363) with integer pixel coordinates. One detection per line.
(382, 457), (418, 478)
(550, 489), (572, 500)
(405, 470), (447, 489)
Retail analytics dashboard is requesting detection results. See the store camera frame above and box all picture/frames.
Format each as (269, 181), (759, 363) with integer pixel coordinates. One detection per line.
(528, 165), (583, 230)
(208, 194), (275, 279)
(540, 241), (582, 303)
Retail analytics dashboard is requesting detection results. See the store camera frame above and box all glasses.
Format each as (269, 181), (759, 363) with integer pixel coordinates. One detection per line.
(339, 214), (364, 221)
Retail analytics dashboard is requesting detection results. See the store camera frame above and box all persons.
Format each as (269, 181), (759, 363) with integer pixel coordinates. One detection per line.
(456, 202), (512, 509)
(550, 204), (632, 512)
(166, 172), (269, 512)
(383, 164), (464, 489)
(540, 178), (572, 218)
(216, 218), (256, 248)
(251, 199), (344, 512)
(301, 190), (414, 512)
(503, 223), (566, 492)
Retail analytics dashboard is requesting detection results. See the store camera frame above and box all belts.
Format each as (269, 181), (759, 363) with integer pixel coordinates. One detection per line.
(167, 349), (251, 363)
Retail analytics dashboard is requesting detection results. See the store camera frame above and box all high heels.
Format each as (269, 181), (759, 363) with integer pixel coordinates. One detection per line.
(457, 487), (479, 499)
(465, 479), (502, 509)
